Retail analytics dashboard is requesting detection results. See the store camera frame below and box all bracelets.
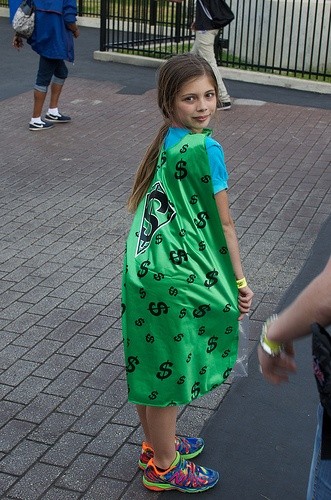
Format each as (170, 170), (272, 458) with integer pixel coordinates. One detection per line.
(72, 27), (78, 33)
(259, 313), (287, 358)
(236, 277), (247, 289)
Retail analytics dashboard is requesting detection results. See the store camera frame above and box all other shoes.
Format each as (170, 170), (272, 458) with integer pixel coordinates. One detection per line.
(216, 101), (232, 111)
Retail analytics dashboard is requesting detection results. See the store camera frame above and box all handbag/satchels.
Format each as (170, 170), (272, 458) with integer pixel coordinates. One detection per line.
(12, 1), (35, 39)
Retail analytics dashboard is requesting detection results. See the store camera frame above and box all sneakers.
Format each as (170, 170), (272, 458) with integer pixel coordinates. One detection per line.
(29, 119), (56, 131)
(142, 451), (220, 494)
(44, 113), (71, 123)
(138, 435), (205, 469)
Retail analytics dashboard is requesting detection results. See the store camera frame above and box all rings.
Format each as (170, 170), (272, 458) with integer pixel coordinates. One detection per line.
(15, 42), (17, 43)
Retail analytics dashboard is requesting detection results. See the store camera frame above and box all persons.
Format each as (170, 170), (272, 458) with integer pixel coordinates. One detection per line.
(12, 0), (80, 130)
(120, 54), (255, 494)
(189, 0), (231, 111)
(257, 261), (331, 500)
